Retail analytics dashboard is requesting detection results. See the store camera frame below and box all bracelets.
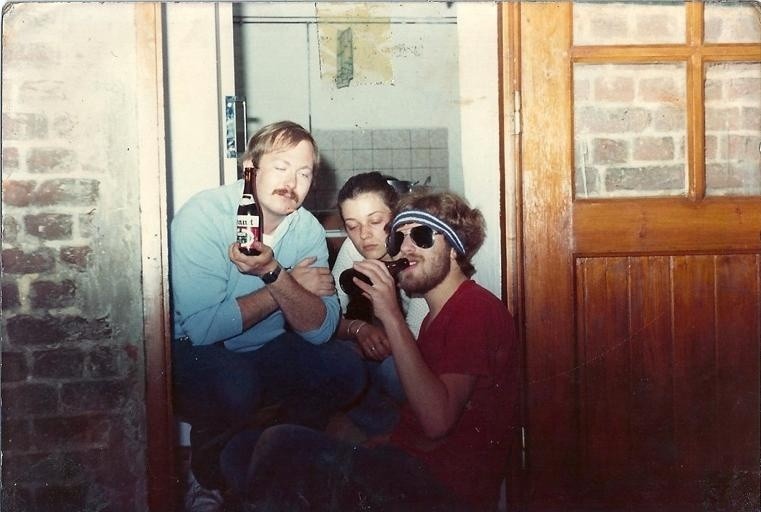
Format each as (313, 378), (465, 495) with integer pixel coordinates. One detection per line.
(345, 319), (358, 336)
(352, 321), (368, 337)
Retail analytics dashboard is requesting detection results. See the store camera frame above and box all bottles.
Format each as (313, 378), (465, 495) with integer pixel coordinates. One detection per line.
(338, 256), (410, 297)
(236, 158), (264, 256)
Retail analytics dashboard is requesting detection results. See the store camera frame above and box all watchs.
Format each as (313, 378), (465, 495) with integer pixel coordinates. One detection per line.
(260, 262), (281, 286)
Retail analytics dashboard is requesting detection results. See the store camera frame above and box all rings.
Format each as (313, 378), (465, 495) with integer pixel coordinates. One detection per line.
(369, 346), (376, 352)
(378, 335), (387, 343)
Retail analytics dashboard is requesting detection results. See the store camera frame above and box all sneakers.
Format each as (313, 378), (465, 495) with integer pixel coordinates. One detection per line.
(184, 476), (226, 511)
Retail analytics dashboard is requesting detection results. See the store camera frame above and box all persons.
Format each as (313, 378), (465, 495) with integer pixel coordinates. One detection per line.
(212, 189), (524, 512)
(166, 118), (371, 512)
(329, 171), (428, 451)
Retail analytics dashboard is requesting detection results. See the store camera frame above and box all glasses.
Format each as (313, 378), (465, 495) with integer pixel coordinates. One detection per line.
(383, 225), (443, 257)
